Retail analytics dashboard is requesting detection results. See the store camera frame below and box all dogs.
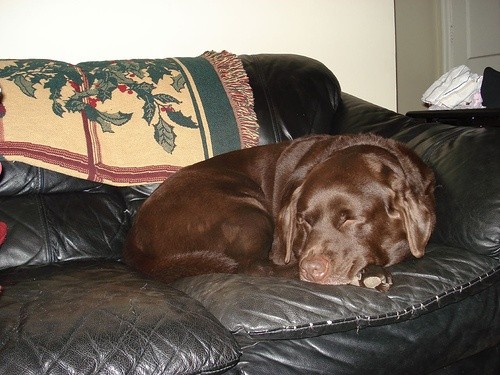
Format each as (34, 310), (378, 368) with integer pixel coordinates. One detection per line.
(121, 132), (437, 291)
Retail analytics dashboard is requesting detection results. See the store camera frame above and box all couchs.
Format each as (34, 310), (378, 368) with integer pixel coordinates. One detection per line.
(1, 49), (500, 375)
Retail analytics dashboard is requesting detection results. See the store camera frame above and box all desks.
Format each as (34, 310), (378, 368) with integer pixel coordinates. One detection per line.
(405, 108), (499, 130)
